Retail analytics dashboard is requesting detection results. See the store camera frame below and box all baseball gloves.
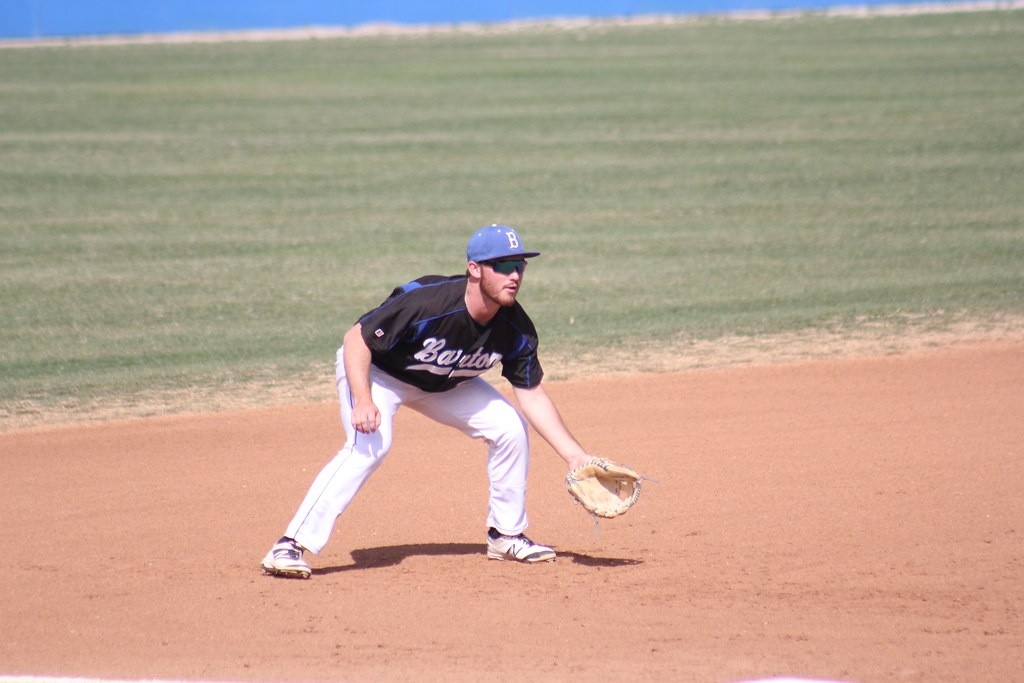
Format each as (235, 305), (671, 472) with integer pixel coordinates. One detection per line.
(561, 457), (660, 527)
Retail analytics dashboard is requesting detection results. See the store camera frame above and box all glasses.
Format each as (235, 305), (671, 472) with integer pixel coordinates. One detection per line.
(476, 260), (528, 274)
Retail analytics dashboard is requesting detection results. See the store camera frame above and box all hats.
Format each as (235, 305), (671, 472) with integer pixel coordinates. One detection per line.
(467, 225), (541, 264)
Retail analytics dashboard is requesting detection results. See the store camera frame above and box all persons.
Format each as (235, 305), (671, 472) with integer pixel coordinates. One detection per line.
(262, 223), (643, 578)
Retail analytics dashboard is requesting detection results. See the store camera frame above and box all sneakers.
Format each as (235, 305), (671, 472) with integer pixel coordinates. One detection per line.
(486, 531), (557, 565)
(260, 537), (313, 580)
